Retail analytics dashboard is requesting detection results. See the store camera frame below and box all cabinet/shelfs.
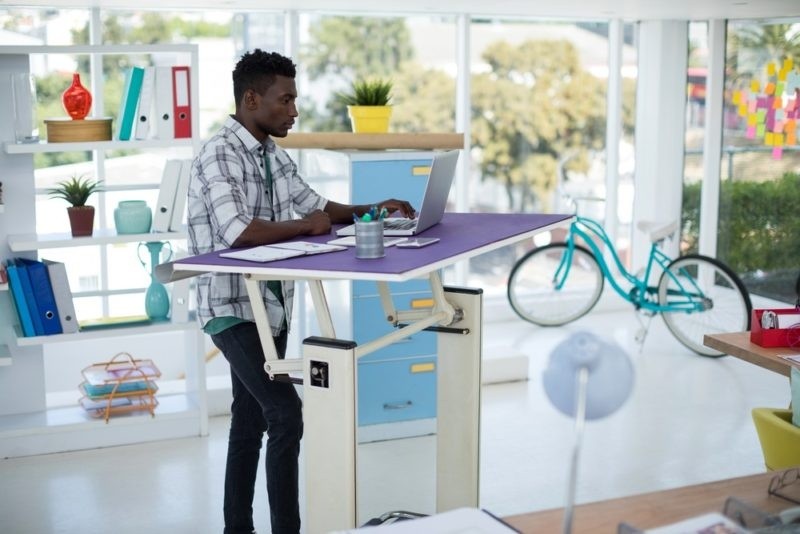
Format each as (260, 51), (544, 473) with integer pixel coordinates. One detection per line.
(0, 42), (210, 460)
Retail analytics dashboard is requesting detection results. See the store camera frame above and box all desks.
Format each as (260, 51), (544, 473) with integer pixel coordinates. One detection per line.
(171, 212), (578, 534)
(703, 329), (800, 378)
(501, 466), (800, 534)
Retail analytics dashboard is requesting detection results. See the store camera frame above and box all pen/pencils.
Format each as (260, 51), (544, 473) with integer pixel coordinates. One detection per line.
(353, 205), (387, 222)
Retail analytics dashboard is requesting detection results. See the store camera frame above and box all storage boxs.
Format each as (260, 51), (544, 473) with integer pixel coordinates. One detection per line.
(751, 308), (800, 349)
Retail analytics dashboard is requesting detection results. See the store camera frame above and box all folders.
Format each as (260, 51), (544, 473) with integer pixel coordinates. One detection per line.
(170, 159), (193, 232)
(172, 67), (192, 138)
(118, 66), (144, 140)
(156, 66), (174, 140)
(13, 258), (45, 336)
(41, 259), (80, 333)
(151, 159), (182, 233)
(21, 258), (62, 335)
(5, 259), (36, 337)
(134, 66), (156, 139)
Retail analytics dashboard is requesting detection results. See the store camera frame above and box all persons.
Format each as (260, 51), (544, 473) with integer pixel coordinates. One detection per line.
(185, 50), (416, 534)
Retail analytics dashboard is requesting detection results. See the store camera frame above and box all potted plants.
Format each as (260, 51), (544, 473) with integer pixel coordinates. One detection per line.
(46, 172), (109, 238)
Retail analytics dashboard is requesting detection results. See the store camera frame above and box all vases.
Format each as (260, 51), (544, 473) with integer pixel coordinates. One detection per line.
(114, 200), (153, 235)
(136, 240), (174, 323)
(751, 407), (800, 471)
(332, 77), (396, 152)
(62, 73), (93, 121)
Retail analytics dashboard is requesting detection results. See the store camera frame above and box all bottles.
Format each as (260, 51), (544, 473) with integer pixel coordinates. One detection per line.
(62, 73), (92, 120)
(136, 241), (173, 321)
(114, 200), (152, 234)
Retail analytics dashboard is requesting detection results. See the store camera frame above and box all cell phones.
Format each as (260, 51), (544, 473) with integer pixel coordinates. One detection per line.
(397, 238), (440, 249)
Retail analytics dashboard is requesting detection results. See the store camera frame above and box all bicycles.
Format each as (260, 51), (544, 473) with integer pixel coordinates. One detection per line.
(506, 150), (753, 358)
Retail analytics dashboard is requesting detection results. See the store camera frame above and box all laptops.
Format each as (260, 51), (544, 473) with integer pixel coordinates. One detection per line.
(336, 149), (461, 236)
